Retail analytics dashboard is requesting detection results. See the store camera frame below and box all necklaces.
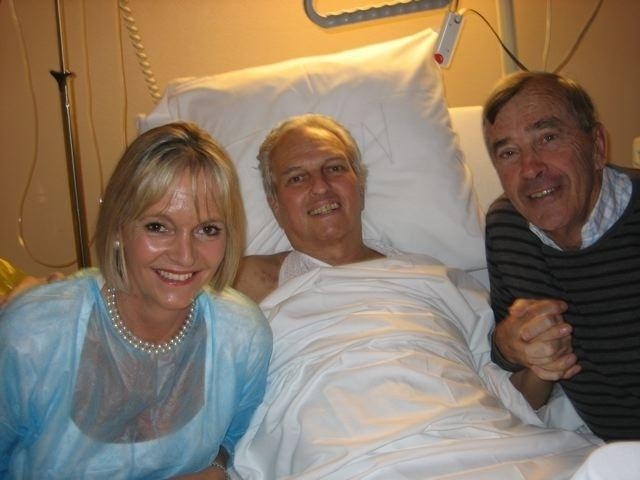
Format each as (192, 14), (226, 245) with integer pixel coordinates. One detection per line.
(104, 283), (201, 358)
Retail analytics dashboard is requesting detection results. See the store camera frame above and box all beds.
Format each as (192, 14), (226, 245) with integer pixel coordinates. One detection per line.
(134, 107), (640, 441)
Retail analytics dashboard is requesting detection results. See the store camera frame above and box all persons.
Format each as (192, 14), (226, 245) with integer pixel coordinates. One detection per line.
(1, 118), (272, 480)
(216, 114), (599, 480)
(479, 73), (639, 447)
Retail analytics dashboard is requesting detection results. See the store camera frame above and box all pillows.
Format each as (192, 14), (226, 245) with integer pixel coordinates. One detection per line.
(168, 26), (485, 274)
